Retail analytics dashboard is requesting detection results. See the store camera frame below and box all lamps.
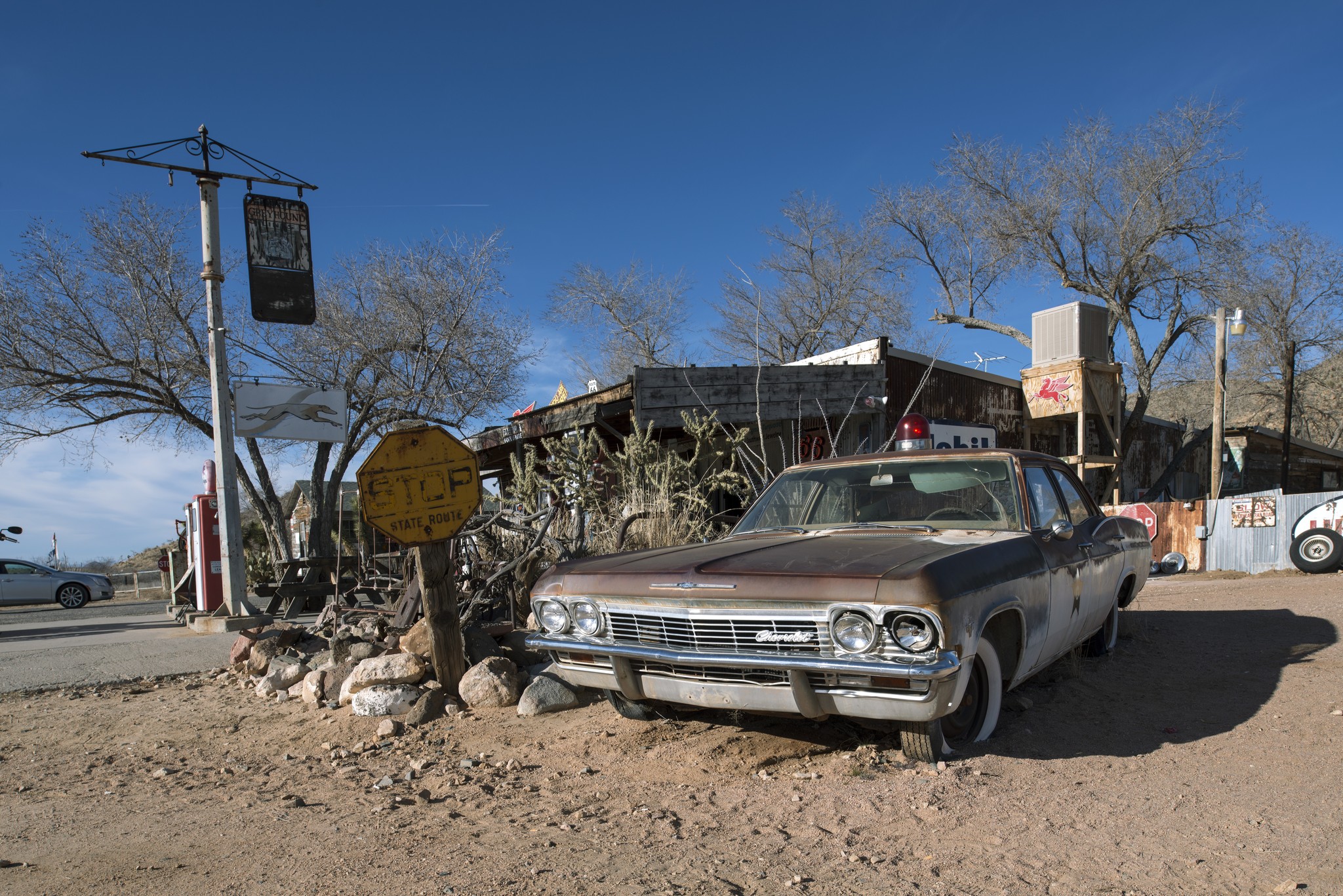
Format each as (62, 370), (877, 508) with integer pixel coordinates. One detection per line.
(865, 395), (888, 407)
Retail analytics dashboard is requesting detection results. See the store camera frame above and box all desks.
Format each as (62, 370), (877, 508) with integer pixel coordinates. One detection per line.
(264, 556), (386, 620)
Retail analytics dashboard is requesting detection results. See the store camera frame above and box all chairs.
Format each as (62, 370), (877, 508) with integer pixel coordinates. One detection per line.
(855, 491), (900, 522)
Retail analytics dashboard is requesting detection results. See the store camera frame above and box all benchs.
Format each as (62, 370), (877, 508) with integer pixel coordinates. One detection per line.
(250, 582), (307, 598)
(339, 577), (395, 593)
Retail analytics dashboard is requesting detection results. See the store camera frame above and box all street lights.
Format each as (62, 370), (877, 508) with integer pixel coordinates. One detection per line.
(1211, 306), (1249, 499)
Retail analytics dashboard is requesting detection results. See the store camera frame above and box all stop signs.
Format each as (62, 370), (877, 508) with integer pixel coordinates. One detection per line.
(356, 425), (484, 550)
(1117, 503), (1157, 542)
(158, 555), (170, 573)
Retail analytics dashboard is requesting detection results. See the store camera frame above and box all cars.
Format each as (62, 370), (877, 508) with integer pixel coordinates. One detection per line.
(0, 558), (116, 609)
(525, 413), (1151, 763)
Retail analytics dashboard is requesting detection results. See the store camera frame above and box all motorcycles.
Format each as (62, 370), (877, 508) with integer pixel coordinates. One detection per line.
(0, 526), (22, 543)
